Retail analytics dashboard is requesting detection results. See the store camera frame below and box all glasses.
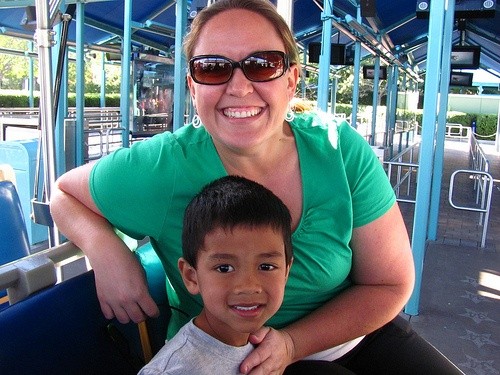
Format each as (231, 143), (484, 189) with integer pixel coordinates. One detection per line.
(187, 50), (290, 85)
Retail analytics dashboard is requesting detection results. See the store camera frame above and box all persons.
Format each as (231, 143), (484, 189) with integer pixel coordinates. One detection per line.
(135, 175), (295, 375)
(46, 0), (466, 375)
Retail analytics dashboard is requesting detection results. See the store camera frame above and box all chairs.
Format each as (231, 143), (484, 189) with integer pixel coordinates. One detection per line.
(1, 269), (163, 375)
(0, 180), (33, 313)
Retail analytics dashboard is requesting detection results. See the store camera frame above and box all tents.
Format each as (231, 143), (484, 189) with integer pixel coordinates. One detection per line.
(1, 0), (500, 318)
